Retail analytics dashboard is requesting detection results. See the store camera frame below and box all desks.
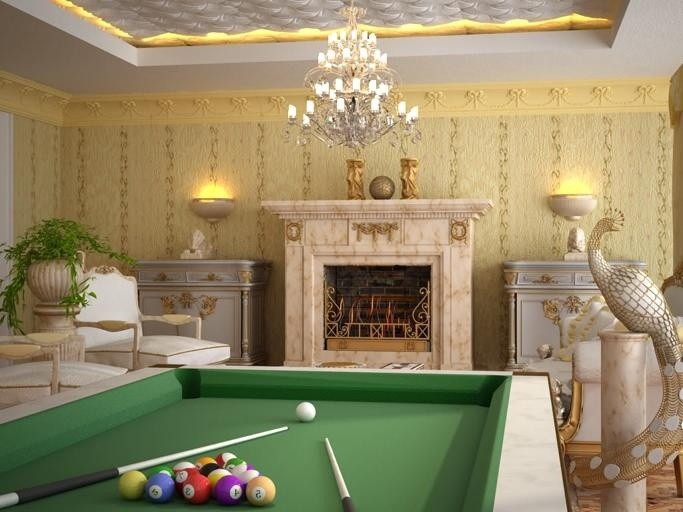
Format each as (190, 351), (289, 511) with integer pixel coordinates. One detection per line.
(1, 362), (575, 512)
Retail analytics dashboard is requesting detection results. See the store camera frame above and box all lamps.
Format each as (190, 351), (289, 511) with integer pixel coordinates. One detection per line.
(284, 0), (425, 159)
(188, 199), (237, 223)
(546, 192), (598, 225)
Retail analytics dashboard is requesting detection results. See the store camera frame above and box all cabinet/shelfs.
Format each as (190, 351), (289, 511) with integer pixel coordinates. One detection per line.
(130, 259), (272, 370)
(501, 260), (649, 370)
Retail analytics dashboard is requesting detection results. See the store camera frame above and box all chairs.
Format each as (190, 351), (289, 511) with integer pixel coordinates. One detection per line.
(75, 269), (231, 368)
(545, 333), (682, 497)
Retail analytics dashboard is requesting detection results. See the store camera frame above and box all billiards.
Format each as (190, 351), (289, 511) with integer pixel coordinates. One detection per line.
(116, 452), (276, 508)
(294, 401), (317, 423)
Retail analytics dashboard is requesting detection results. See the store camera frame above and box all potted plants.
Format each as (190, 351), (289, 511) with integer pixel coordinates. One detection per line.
(0, 211), (132, 337)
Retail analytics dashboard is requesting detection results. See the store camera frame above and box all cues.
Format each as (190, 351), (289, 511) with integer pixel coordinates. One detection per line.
(324, 436), (358, 512)
(0, 426), (289, 510)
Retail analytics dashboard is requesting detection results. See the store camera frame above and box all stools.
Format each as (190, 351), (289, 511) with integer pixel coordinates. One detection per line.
(0, 332), (127, 410)
